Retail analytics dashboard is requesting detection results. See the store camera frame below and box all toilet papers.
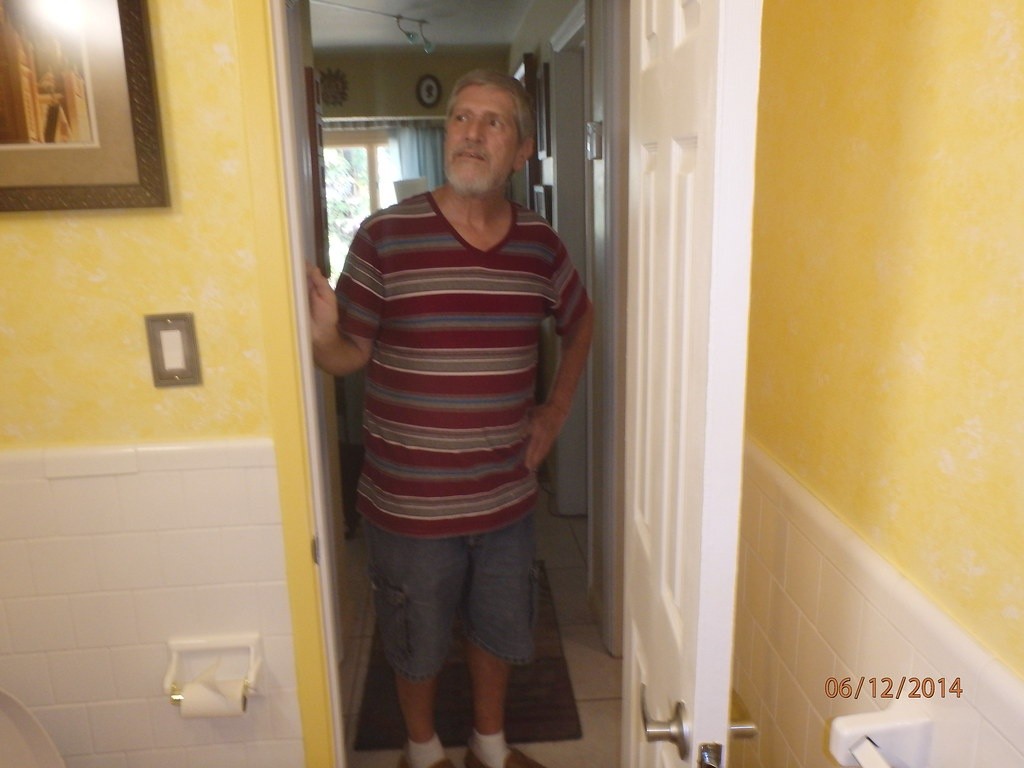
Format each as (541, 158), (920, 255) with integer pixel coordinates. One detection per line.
(179, 672), (252, 720)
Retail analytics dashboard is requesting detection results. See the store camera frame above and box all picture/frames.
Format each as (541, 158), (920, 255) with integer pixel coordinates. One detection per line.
(2, 0), (174, 216)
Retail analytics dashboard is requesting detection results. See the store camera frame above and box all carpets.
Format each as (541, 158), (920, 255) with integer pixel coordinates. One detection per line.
(352, 559), (583, 753)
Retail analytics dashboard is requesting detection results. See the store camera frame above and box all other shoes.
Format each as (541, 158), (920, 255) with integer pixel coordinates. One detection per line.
(396, 744), (549, 768)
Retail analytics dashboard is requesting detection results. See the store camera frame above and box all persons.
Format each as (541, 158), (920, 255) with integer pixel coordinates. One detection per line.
(305, 69), (595, 768)
